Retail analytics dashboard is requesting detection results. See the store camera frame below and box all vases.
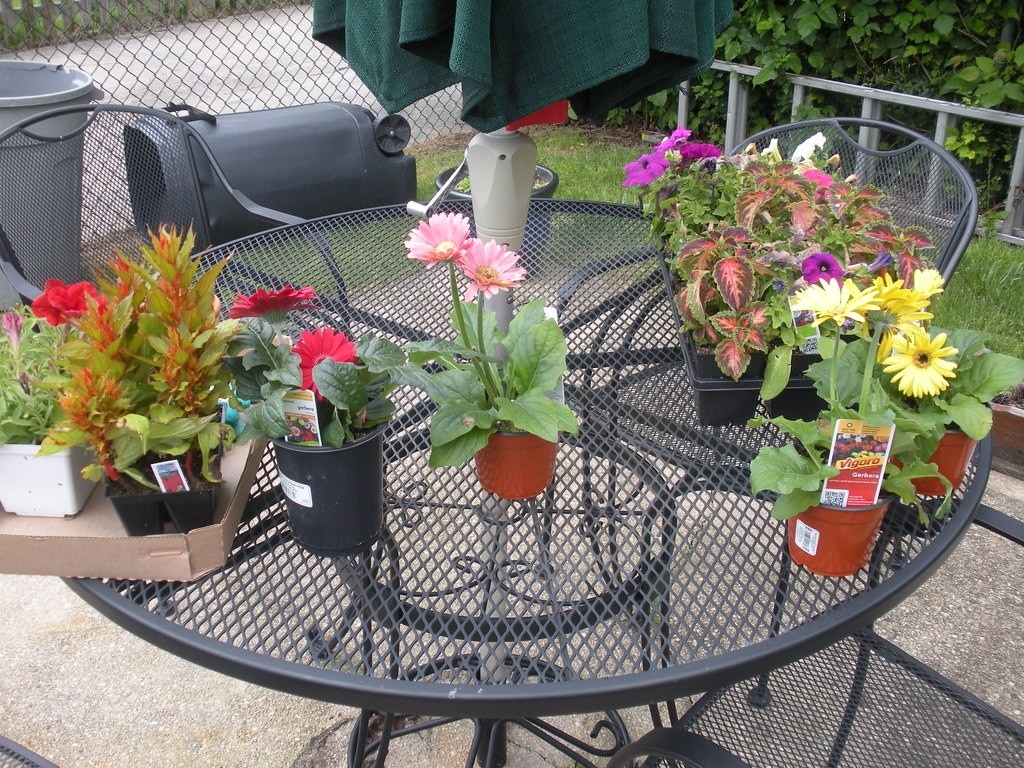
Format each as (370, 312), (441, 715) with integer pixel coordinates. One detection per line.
(268, 418), (388, 558)
(0, 444), (98, 519)
(780, 495), (897, 577)
(436, 164), (560, 278)
(475, 432), (559, 503)
(885, 402), (992, 496)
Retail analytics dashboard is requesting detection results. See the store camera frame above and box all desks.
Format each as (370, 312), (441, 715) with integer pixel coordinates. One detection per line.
(70, 198), (999, 768)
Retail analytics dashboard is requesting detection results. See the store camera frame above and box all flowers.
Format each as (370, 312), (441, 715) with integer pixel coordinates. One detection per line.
(806, 263), (1024, 438)
(407, 212), (580, 465)
(228, 283), (507, 449)
(746, 272), (995, 530)
(621, 125), (938, 383)
(0, 276), (107, 444)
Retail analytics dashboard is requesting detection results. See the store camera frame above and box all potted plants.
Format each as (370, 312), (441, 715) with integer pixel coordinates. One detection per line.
(654, 243), (884, 427)
(42, 229), (253, 539)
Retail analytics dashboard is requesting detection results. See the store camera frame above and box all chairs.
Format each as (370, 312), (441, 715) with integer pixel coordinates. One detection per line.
(610, 497), (1024, 768)
(541, 115), (983, 710)
